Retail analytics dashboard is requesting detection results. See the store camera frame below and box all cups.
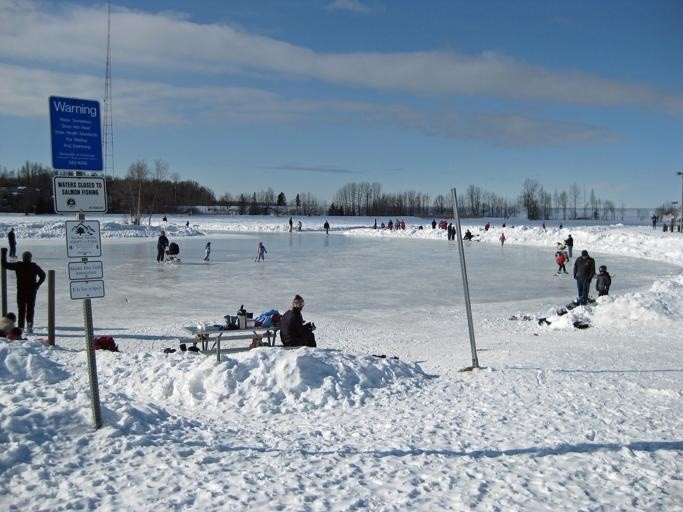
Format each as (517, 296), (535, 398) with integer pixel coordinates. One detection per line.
(246, 318), (255, 328)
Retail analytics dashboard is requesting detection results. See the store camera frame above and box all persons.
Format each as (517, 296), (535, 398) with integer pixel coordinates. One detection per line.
(381, 218), (405, 230)
(542, 224), (612, 306)
(298, 220), (302, 231)
(324, 220), (329, 234)
(156, 231), (169, 264)
(432, 219), (456, 239)
(202, 242), (210, 260)
(650, 214), (681, 233)
(186, 221), (189, 226)
(163, 216), (167, 221)
(0, 227), (46, 341)
(464, 222), (506, 247)
(289, 217), (293, 232)
(279, 294), (317, 347)
(257, 242), (267, 263)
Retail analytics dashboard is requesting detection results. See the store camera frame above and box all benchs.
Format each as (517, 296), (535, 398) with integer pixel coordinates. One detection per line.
(200, 344), (303, 365)
(176, 332), (277, 349)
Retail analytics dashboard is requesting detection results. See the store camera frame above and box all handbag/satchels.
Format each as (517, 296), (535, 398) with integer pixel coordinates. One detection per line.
(95, 337), (118, 350)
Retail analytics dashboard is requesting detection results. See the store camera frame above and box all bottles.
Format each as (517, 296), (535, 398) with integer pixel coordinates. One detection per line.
(236, 305), (246, 330)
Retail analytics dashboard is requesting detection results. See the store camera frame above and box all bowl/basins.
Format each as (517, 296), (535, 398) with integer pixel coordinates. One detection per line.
(223, 315), (238, 326)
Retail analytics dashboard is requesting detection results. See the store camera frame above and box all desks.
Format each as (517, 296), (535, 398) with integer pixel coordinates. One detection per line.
(181, 324), (280, 363)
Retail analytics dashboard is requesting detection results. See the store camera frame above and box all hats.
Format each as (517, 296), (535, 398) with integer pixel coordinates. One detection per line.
(292, 295), (304, 308)
